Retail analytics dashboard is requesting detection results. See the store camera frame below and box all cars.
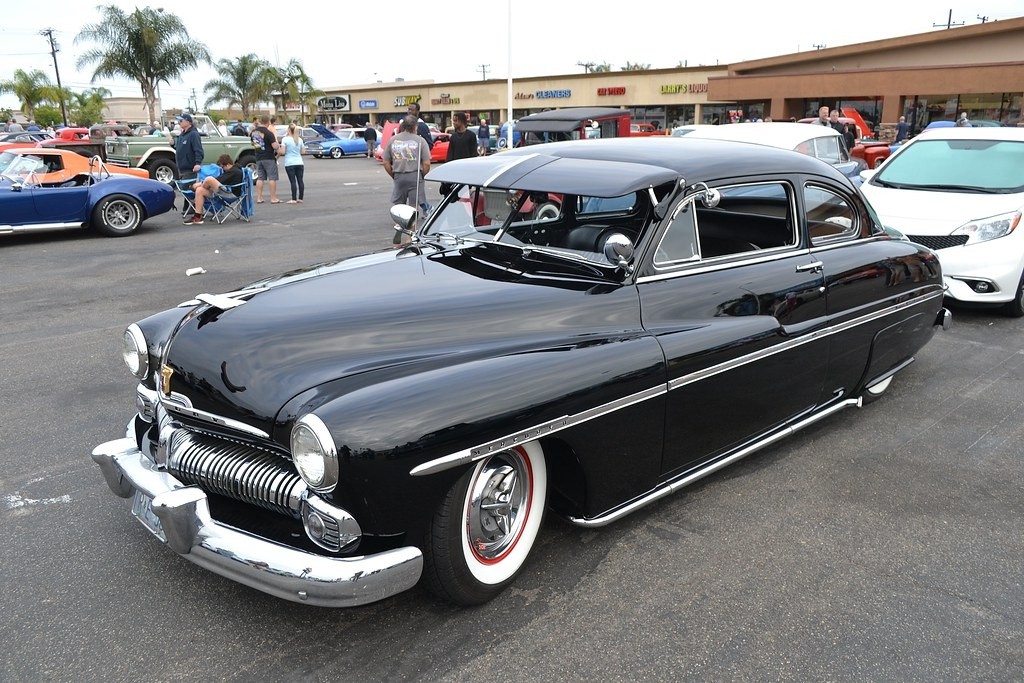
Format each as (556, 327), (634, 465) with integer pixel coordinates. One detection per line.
(792, 115), (1024, 169)
(0, 105), (714, 163)
(681, 121), (873, 205)
(0, 147), (150, 183)
(825, 127), (1024, 314)
(0, 154), (176, 236)
(90, 137), (940, 611)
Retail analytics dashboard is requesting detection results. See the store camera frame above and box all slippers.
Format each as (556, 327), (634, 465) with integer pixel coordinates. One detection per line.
(257, 200), (264, 203)
(271, 199), (284, 204)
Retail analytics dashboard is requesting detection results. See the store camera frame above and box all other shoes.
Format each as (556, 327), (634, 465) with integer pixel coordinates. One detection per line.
(367, 156), (375, 159)
(276, 161), (280, 166)
(286, 199), (303, 204)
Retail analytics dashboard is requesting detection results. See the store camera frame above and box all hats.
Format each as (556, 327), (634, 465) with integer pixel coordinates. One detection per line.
(842, 121), (850, 127)
(176, 113), (193, 123)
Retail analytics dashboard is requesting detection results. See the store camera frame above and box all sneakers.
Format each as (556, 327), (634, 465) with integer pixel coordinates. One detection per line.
(393, 224), (403, 231)
(183, 216), (204, 225)
(410, 231), (418, 241)
(422, 207), (435, 219)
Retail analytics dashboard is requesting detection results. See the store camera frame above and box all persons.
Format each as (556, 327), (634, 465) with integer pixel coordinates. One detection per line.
(247, 117), (262, 136)
(267, 118), (281, 165)
(398, 102), (434, 220)
(170, 113), (204, 214)
(383, 114), (431, 245)
(478, 119), (490, 156)
(495, 122), (504, 140)
(232, 119), (246, 135)
(216, 119), (232, 136)
(278, 123), (306, 204)
(250, 115), (285, 204)
(0, 117), (169, 138)
(731, 100), (981, 179)
(183, 153), (244, 226)
(359, 121), (383, 159)
(446, 112), (477, 162)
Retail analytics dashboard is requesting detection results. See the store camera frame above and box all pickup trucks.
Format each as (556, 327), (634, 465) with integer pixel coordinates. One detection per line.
(104, 112), (264, 184)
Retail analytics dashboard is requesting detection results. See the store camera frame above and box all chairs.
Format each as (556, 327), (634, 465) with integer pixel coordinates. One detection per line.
(174, 163), (223, 219)
(210, 167), (253, 224)
